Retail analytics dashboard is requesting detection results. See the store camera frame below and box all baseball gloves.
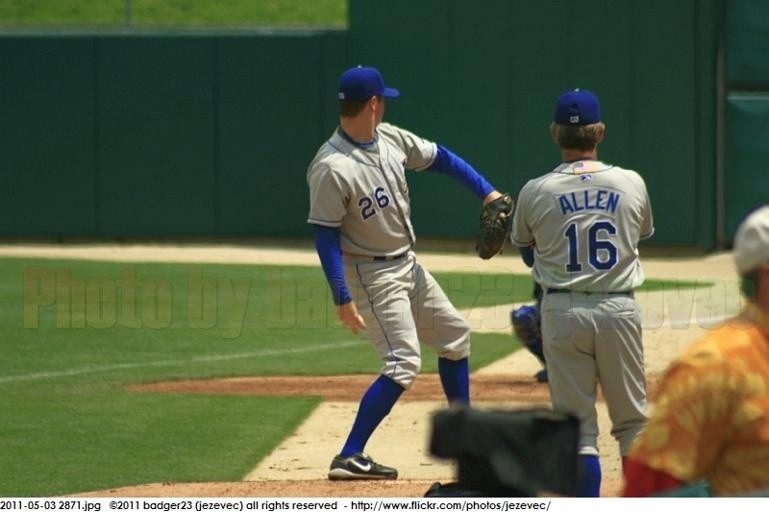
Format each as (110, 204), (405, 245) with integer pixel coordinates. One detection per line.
(475, 193), (514, 261)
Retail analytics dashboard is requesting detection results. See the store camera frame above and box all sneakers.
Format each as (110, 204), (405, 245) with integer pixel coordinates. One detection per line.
(327, 452), (398, 480)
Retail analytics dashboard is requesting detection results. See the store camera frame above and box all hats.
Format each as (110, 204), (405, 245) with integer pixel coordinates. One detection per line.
(732, 204), (769, 274)
(555, 88), (602, 126)
(337, 65), (400, 102)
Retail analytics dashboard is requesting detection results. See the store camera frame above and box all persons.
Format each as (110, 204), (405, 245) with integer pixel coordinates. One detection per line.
(306, 66), (515, 481)
(510, 91), (656, 497)
(615, 204), (769, 496)
(511, 284), (547, 383)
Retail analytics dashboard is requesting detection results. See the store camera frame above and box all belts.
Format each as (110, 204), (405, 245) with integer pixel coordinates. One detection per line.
(547, 288), (630, 295)
(374, 250), (408, 262)
